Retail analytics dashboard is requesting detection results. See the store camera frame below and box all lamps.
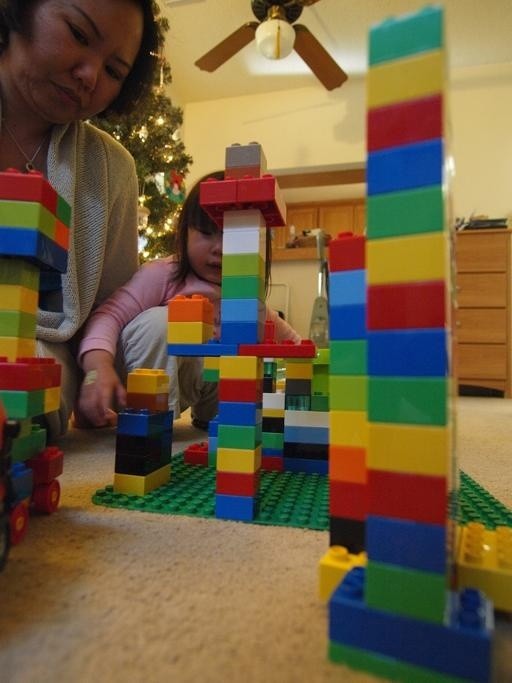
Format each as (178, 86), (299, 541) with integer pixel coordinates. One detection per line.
(255, 5), (296, 60)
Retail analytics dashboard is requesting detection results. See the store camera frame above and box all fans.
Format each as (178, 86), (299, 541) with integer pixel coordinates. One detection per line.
(195, 0), (347, 91)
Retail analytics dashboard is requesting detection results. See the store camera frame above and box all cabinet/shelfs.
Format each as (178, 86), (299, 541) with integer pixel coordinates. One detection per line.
(455, 229), (512, 398)
(271, 199), (366, 261)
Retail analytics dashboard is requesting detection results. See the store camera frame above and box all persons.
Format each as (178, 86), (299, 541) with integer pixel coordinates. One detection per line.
(75, 168), (301, 428)
(1, 0), (159, 438)
